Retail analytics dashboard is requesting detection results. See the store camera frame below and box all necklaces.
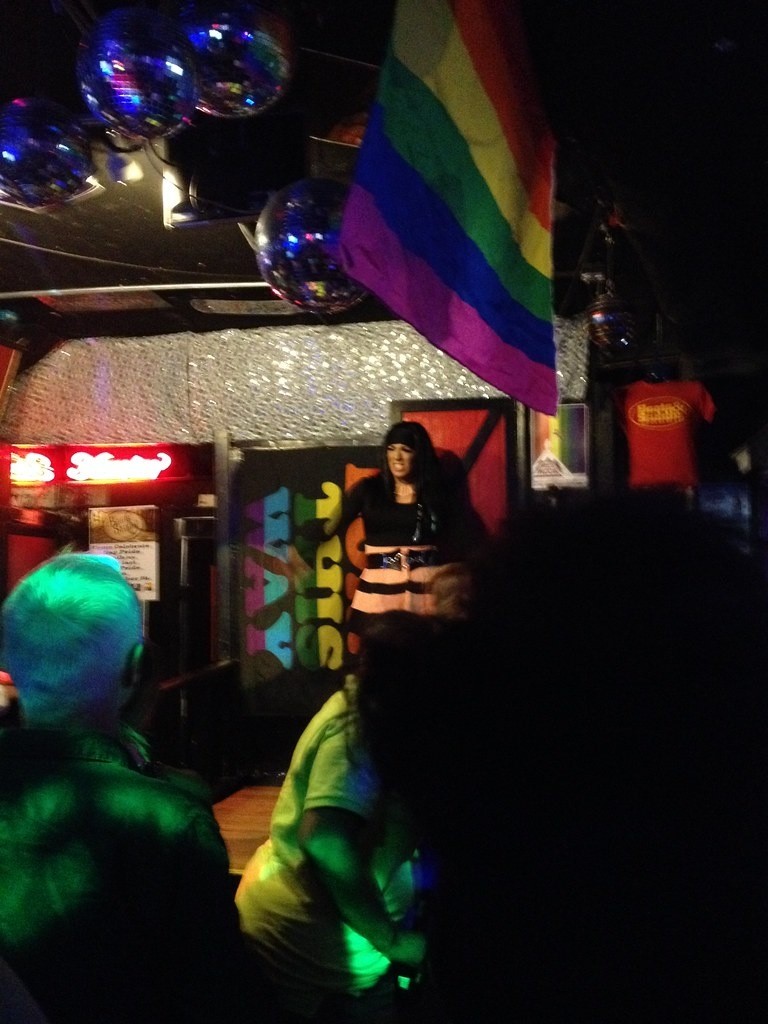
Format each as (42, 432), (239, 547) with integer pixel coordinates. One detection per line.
(393, 487), (418, 497)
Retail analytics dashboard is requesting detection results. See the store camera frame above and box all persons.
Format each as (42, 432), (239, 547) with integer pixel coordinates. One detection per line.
(321, 418), (494, 674)
(609, 362), (719, 517)
(2, 551), (251, 1024)
(235, 609), (456, 1024)
(345, 488), (768, 1022)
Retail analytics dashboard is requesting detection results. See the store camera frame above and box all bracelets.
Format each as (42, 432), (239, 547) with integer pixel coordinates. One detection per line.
(372, 923), (400, 954)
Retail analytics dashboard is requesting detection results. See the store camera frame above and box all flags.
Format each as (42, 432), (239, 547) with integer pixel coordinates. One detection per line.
(328, 3), (559, 422)
